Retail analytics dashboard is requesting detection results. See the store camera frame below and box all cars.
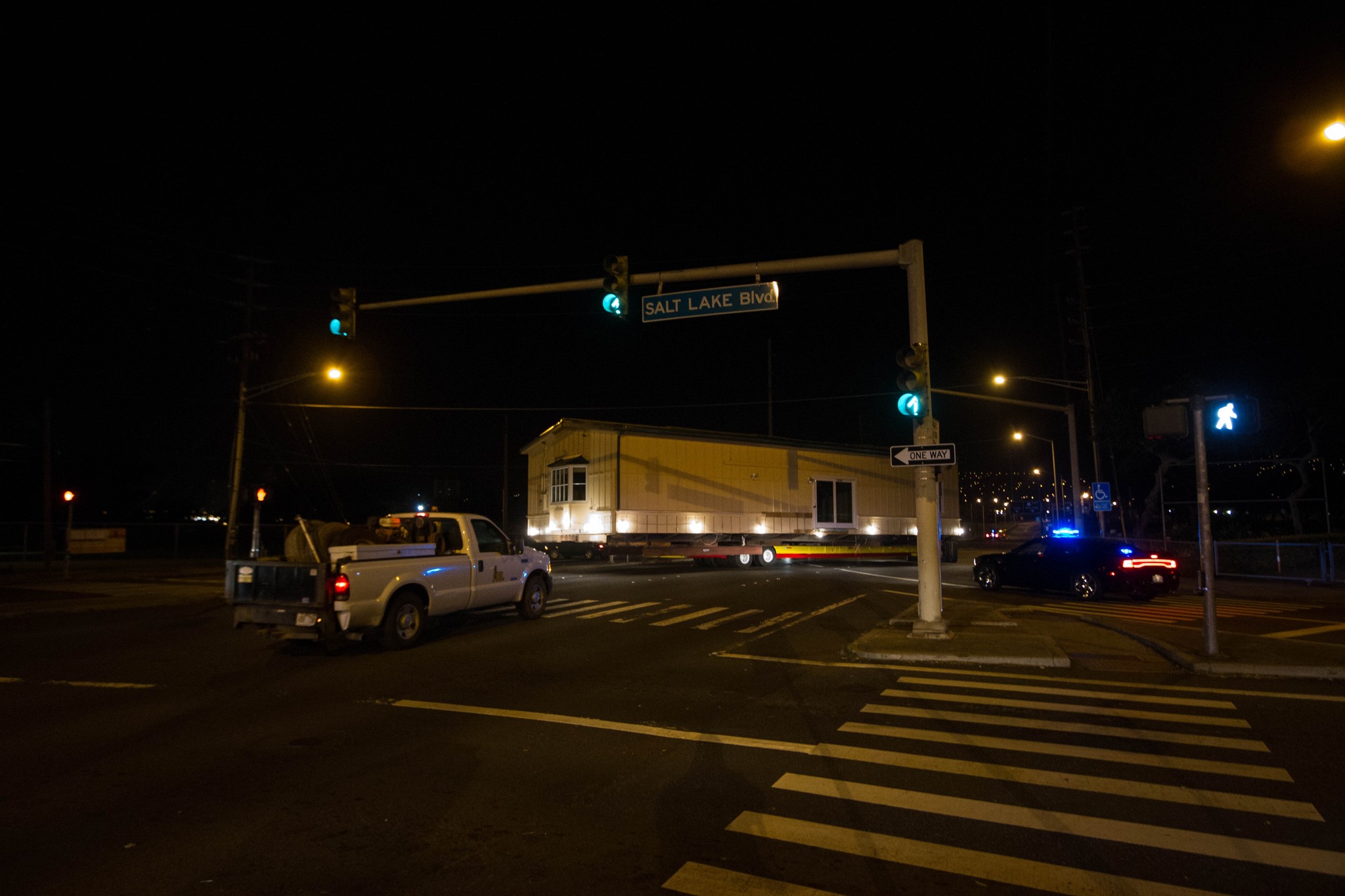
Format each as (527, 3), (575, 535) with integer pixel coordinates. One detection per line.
(971, 527), (1180, 602)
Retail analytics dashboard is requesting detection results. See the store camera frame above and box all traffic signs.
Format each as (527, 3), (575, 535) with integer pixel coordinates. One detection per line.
(890, 442), (957, 467)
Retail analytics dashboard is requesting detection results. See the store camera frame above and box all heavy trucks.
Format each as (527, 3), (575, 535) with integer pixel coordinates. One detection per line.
(519, 415), (963, 572)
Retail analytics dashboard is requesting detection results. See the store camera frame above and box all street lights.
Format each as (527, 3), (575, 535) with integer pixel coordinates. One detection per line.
(1033, 469), (1066, 525)
(977, 499), (985, 539)
(1004, 502), (1011, 526)
(1014, 432), (1060, 535)
(994, 498), (998, 532)
(992, 374), (1105, 538)
(222, 366), (343, 572)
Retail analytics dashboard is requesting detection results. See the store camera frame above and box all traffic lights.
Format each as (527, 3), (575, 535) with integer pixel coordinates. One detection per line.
(256, 487), (267, 502)
(1212, 399), (1260, 435)
(895, 342), (930, 418)
(602, 254), (629, 317)
(329, 287), (357, 339)
(64, 491), (75, 501)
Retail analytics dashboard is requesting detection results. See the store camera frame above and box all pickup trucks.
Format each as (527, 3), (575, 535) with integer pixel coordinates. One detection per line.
(221, 510), (555, 651)
